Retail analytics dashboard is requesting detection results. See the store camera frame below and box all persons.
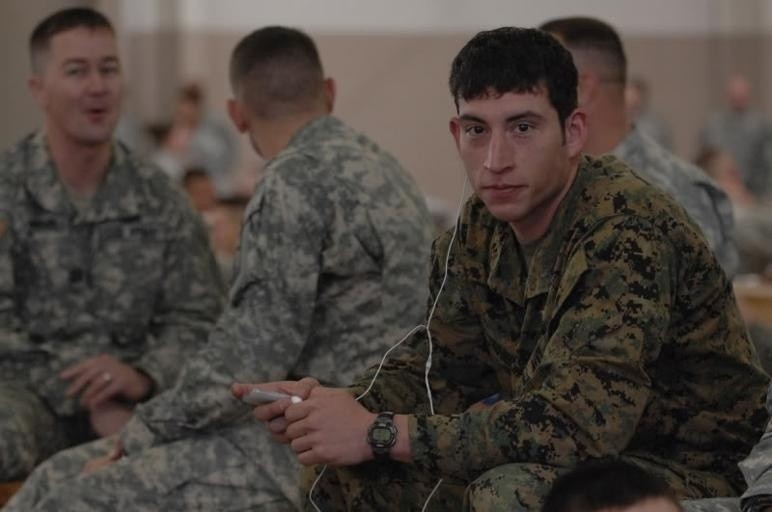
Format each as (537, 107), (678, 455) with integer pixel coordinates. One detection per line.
(228, 25), (771, 511)
(2, 25), (437, 512)
(536, 460), (685, 511)
(624, 79), (661, 145)
(2, 8), (228, 482)
(140, 84), (266, 289)
(693, 143), (772, 278)
(538, 16), (741, 285)
(709, 71), (772, 192)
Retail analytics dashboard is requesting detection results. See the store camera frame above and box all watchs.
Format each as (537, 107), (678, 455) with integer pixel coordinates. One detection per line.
(366, 408), (398, 462)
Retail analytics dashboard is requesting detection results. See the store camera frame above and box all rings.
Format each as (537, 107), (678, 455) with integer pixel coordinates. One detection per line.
(101, 370), (113, 384)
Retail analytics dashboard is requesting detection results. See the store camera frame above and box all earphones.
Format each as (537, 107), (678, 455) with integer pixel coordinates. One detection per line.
(292, 396), (302, 404)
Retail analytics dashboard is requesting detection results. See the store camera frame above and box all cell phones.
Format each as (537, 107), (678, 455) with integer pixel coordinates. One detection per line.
(243, 388), (291, 406)
